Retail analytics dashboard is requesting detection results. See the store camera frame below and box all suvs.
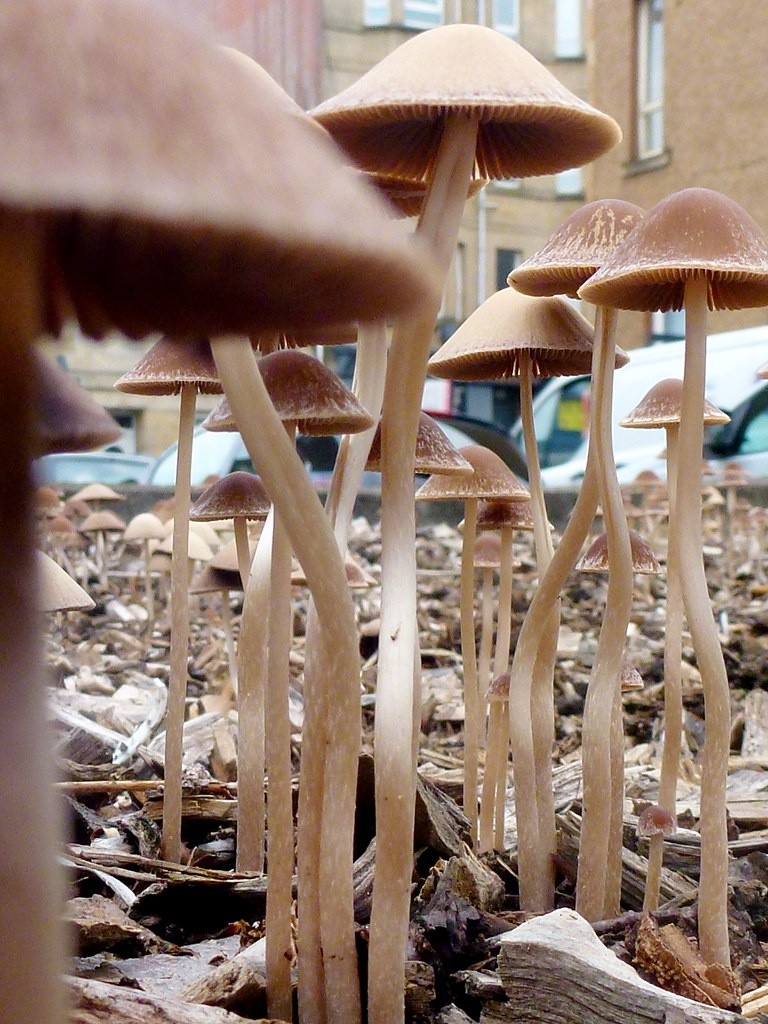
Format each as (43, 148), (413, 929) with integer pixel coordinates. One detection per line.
(41, 408), (571, 540)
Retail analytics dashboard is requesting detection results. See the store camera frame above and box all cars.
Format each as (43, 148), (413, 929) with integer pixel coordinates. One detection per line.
(614, 383), (768, 504)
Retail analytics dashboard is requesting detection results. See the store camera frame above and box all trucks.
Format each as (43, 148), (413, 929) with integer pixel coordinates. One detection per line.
(505, 327), (768, 488)
